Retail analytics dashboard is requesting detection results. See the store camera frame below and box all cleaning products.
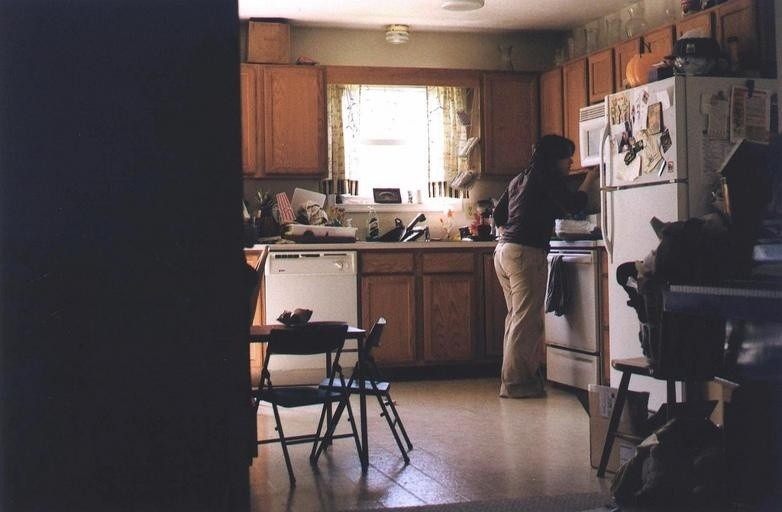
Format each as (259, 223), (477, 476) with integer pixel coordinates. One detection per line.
(446, 208), (461, 241)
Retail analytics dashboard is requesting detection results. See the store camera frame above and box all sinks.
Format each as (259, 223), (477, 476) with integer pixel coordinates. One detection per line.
(413, 234), (444, 242)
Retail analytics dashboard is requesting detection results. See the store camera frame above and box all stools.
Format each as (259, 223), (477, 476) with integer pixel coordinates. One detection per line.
(593, 355), (715, 478)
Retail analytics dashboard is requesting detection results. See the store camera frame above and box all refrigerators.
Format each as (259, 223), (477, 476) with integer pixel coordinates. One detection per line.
(266, 250), (360, 388)
(599, 75), (781, 414)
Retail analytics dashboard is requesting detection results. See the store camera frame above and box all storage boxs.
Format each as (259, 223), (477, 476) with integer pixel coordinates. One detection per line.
(586, 383), (650, 476)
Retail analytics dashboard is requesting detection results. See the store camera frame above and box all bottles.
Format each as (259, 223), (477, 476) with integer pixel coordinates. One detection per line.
(727, 36), (741, 76)
(553, 2), (647, 65)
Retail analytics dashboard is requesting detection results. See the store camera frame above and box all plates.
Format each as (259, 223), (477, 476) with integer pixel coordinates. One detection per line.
(554, 219), (590, 240)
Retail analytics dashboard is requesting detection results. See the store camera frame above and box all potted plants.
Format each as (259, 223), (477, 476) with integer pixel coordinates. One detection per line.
(252, 185), (277, 237)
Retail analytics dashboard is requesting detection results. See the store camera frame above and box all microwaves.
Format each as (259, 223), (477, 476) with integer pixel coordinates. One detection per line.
(577, 101), (605, 168)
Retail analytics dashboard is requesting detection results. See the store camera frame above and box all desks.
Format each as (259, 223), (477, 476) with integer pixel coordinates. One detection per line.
(659, 282), (782, 511)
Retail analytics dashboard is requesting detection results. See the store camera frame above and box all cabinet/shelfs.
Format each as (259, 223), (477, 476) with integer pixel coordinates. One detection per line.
(242, 62), (329, 180)
(587, 0), (774, 104)
(358, 247), (507, 369)
(465, 70), (540, 179)
(539, 56), (588, 175)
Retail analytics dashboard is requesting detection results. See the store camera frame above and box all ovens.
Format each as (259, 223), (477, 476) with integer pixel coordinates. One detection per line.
(543, 249), (602, 395)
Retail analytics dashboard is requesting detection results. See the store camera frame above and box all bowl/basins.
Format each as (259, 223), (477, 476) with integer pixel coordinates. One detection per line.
(276, 311), (313, 327)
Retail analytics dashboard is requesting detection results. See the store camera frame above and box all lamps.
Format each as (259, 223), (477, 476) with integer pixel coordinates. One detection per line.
(385, 25), (411, 45)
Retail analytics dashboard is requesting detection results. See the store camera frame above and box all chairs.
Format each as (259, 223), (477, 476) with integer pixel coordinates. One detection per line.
(251, 317), (413, 488)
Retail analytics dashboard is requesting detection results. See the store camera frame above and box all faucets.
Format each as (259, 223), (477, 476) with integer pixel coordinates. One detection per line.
(401, 213), (426, 240)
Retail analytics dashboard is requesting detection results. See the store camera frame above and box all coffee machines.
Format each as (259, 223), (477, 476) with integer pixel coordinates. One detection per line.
(473, 200), (497, 242)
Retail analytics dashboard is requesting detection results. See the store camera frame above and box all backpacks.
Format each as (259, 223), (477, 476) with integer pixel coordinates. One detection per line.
(616, 216), (745, 383)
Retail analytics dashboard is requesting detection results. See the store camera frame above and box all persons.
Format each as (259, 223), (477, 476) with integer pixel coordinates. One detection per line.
(493, 134), (597, 398)
